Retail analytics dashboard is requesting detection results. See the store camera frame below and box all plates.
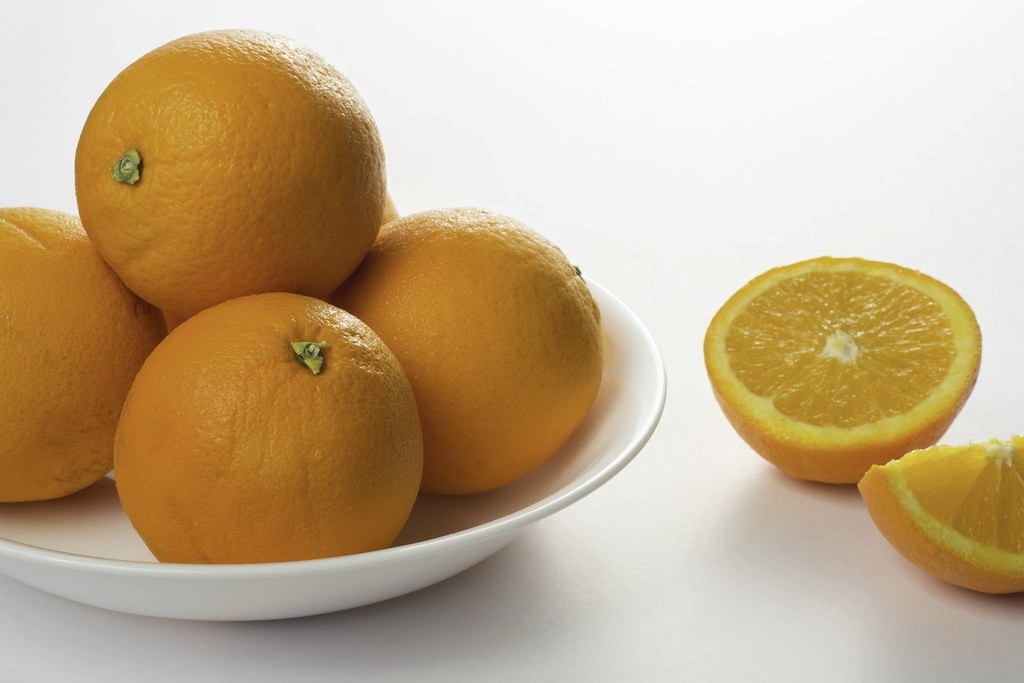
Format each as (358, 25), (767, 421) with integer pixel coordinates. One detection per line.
(0, 273), (667, 622)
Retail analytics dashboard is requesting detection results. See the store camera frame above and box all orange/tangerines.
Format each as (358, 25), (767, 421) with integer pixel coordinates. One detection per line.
(1, 29), (601, 563)
(856, 433), (1024, 601)
(704, 256), (982, 485)
